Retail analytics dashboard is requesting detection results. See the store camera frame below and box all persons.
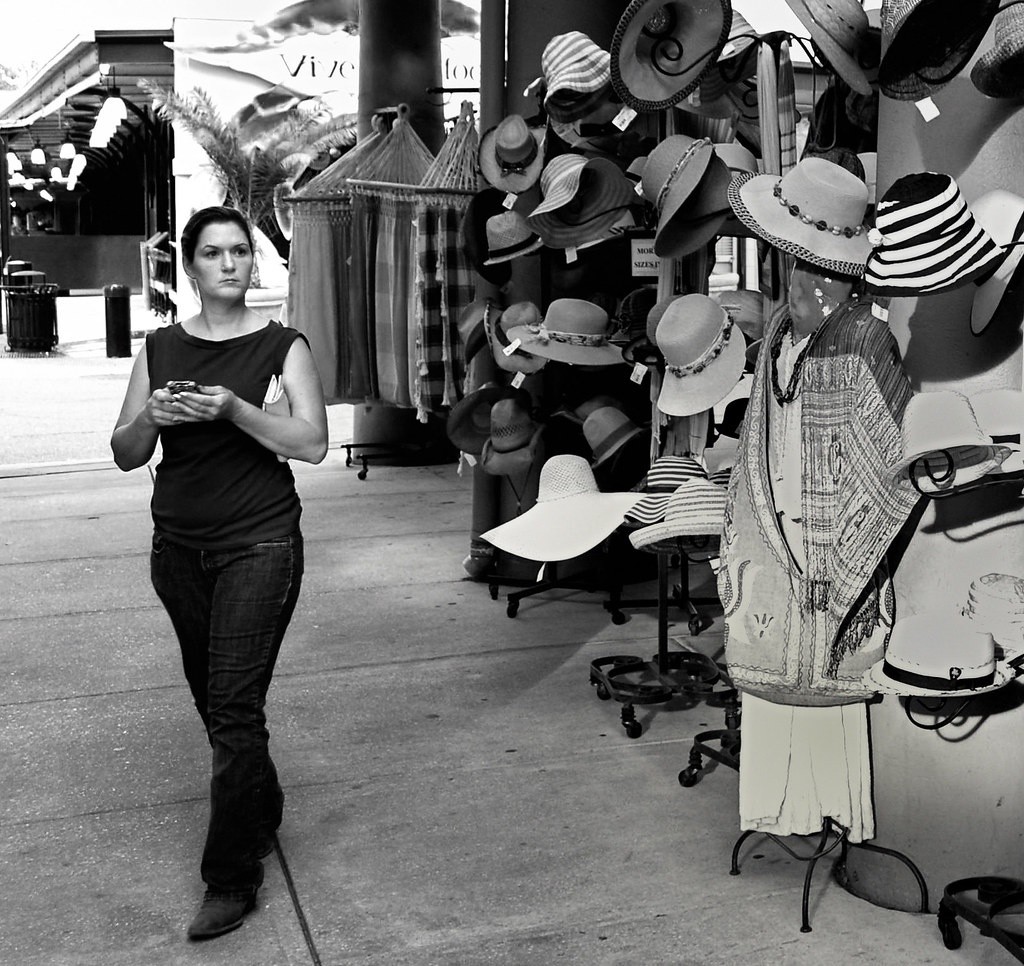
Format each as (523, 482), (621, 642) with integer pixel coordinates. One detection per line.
(466, 189), (582, 312)
(110, 205), (329, 935)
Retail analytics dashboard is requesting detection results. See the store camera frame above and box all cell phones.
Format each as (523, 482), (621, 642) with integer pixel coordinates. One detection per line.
(167, 380), (200, 402)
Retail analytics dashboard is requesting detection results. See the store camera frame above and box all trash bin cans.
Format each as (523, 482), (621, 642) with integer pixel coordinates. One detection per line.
(6, 283), (59, 352)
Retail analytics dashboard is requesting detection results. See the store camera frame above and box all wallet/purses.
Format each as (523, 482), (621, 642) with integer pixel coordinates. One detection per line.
(263, 374), (291, 463)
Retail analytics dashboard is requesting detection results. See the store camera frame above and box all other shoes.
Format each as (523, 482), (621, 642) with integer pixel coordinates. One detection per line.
(250, 803), (282, 861)
(187, 881), (264, 937)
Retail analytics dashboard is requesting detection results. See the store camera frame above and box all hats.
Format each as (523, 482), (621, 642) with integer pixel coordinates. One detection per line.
(802, 147), (866, 183)
(968, 386), (1024, 474)
(784, 0), (873, 96)
(877, 0), (1000, 101)
(882, 390), (1012, 493)
(858, 171), (1006, 298)
(970, 0), (1024, 99)
(861, 613), (1016, 696)
(436, 0), (794, 566)
(845, 84), (879, 136)
(727, 157), (874, 277)
(970, 188), (1024, 337)
(962, 573), (1024, 655)
(810, 26), (881, 91)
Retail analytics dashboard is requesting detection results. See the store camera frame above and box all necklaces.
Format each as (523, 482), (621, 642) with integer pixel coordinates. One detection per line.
(770, 316), (816, 408)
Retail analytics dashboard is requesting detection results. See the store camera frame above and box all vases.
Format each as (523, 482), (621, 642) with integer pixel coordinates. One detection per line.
(243, 282), (290, 322)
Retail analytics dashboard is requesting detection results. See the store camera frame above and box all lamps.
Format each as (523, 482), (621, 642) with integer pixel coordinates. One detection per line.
(60, 130), (76, 158)
(67, 143), (87, 190)
(30, 136), (47, 164)
(51, 162), (61, 178)
(38, 187), (54, 202)
(89, 65), (128, 148)
(6, 144), (25, 185)
